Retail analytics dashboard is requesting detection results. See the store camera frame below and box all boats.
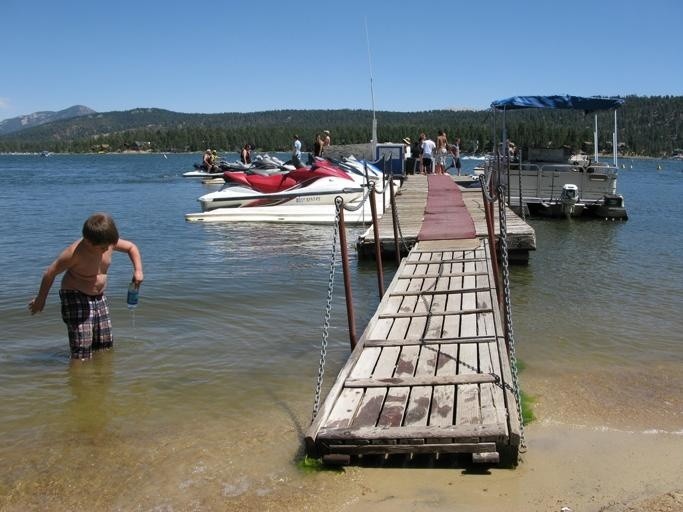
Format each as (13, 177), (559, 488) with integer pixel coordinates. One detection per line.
(484, 94), (630, 222)
(525, 182), (587, 219)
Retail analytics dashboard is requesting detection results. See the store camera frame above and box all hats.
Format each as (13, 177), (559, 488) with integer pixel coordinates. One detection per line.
(403, 137), (411, 145)
(323, 130), (330, 135)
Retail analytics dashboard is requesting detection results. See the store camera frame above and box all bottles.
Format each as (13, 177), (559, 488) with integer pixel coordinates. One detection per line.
(126, 282), (140, 311)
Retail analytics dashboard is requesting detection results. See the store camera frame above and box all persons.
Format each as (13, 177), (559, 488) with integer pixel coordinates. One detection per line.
(314, 133), (323, 156)
(28, 214), (144, 358)
(241, 143), (251, 164)
(323, 130), (330, 146)
(292, 135), (301, 163)
(508, 141), (520, 162)
(203, 149), (219, 173)
(402, 129), (461, 177)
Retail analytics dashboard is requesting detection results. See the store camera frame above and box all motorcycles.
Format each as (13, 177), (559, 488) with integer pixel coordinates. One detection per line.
(180, 154), (401, 224)
